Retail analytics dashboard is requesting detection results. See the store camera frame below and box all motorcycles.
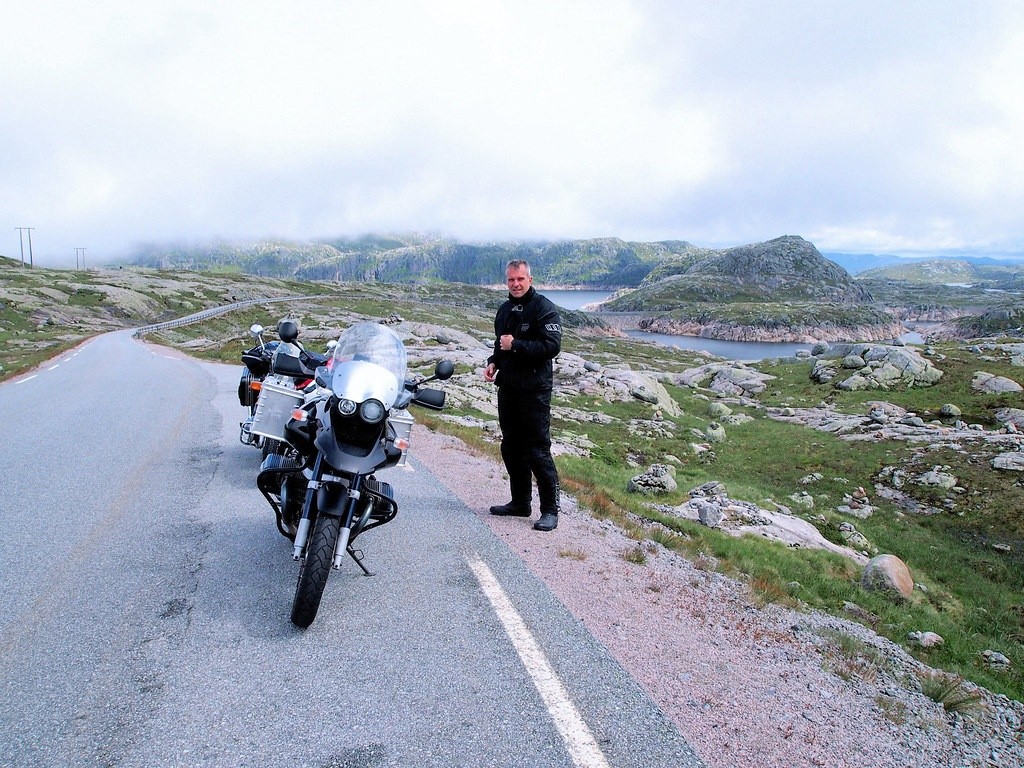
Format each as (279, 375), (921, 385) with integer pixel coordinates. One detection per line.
(239, 320), (454, 629)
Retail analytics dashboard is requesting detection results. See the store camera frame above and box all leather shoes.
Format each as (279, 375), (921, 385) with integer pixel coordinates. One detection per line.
(534, 513), (558, 531)
(490, 501), (531, 517)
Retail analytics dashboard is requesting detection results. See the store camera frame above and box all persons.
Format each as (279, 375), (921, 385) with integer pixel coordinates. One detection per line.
(483, 259), (562, 532)
